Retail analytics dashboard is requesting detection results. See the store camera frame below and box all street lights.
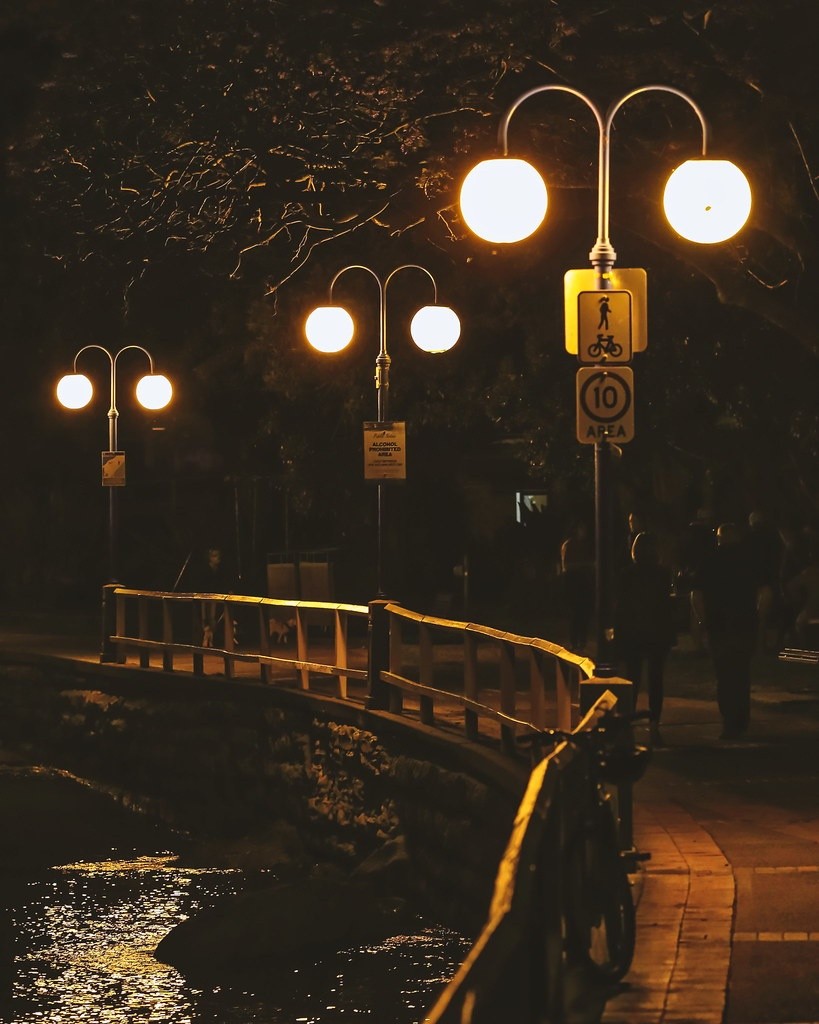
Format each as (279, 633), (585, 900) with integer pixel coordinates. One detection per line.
(56, 345), (175, 664)
(304, 263), (461, 711)
(459, 86), (754, 875)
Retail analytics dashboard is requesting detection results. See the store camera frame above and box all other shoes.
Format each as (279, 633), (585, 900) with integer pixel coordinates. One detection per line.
(719, 730), (741, 743)
(648, 734), (669, 748)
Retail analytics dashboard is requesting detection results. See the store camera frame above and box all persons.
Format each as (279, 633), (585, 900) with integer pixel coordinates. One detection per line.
(200, 548), (233, 647)
(619, 533), (677, 745)
(690, 523), (770, 740)
